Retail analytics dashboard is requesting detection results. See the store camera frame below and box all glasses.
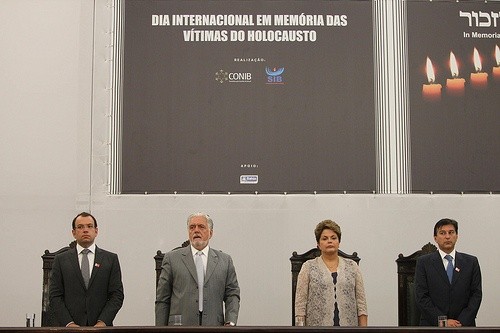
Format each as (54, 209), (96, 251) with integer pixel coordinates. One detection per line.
(74, 225), (95, 230)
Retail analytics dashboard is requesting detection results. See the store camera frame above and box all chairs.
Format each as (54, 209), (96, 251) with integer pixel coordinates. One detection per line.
(395, 242), (438, 326)
(290, 246), (361, 326)
(41, 241), (77, 325)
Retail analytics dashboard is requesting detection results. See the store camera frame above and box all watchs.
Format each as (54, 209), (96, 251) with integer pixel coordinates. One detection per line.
(225, 322), (236, 326)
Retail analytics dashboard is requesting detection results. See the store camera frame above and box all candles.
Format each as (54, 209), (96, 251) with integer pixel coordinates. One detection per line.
(421, 44), (500, 102)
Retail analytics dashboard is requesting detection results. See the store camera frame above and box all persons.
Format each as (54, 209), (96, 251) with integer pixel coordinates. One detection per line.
(295, 220), (368, 326)
(155, 212), (240, 327)
(415, 219), (482, 327)
(48, 212), (124, 327)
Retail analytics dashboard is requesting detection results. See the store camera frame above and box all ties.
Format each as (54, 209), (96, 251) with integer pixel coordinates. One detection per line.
(197, 251), (206, 312)
(444, 255), (453, 286)
(81, 248), (90, 289)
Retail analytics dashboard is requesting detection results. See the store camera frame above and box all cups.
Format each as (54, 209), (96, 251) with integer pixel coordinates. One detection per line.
(438, 316), (448, 327)
(295, 316), (304, 326)
(173, 315), (182, 326)
(26, 312), (35, 328)
